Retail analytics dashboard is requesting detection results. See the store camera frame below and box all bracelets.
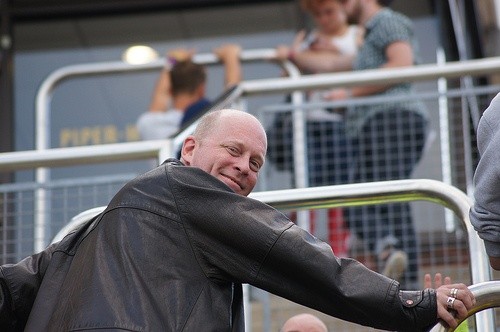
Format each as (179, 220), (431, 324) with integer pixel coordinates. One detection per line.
(288, 48), (294, 61)
(164, 56), (179, 70)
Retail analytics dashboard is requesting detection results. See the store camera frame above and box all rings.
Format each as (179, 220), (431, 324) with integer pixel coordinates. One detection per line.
(451, 288), (457, 298)
(447, 297), (455, 309)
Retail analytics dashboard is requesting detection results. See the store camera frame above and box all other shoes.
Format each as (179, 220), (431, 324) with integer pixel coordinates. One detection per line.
(380, 251), (408, 282)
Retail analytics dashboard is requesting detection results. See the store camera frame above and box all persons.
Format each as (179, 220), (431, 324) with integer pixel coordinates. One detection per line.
(139, 0), (500, 332)
(0, 110), (475, 332)
